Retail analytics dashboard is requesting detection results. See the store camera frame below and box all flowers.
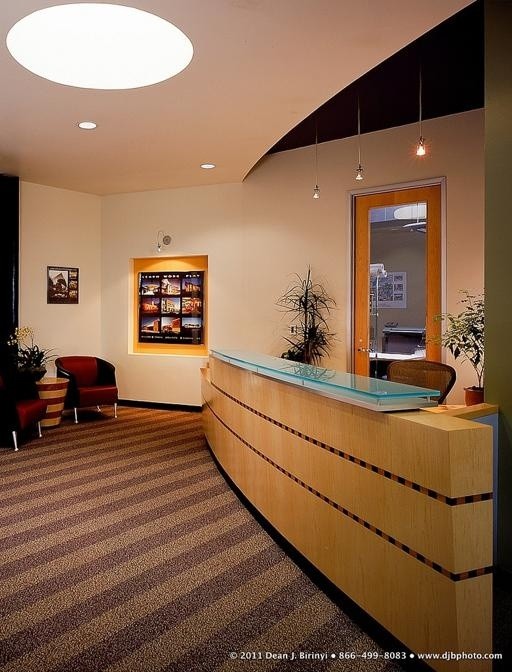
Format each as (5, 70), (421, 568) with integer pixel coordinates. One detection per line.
(7, 324), (63, 369)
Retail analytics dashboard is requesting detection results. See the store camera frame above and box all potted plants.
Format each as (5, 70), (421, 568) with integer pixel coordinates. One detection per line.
(424, 289), (485, 406)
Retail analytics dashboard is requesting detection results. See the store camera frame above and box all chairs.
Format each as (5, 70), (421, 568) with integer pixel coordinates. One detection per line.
(387, 359), (456, 404)
(0, 356), (118, 452)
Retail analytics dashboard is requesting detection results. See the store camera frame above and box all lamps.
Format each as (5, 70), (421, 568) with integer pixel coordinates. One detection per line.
(355, 95), (364, 180)
(416, 63), (426, 156)
(313, 127), (320, 199)
(157, 230), (171, 249)
